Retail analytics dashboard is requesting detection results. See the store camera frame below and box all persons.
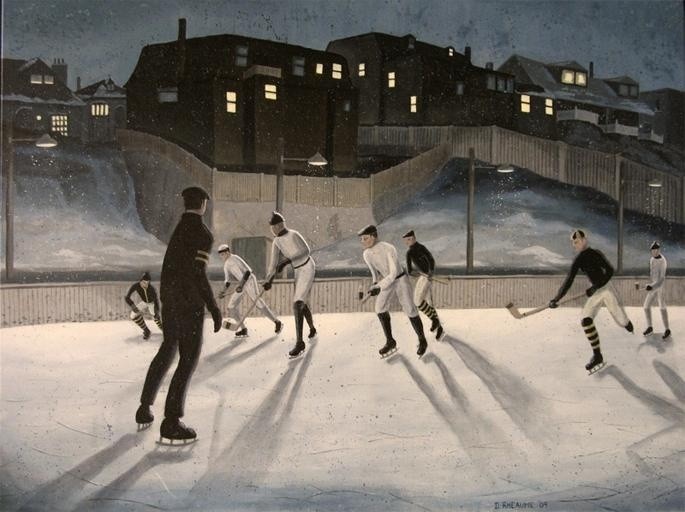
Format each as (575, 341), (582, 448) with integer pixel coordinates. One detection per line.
(136, 187), (222, 441)
(124, 273), (164, 342)
(263, 212), (316, 357)
(549, 230), (633, 369)
(357, 225), (427, 356)
(643, 241), (670, 340)
(403, 229), (442, 340)
(217, 245), (281, 336)
